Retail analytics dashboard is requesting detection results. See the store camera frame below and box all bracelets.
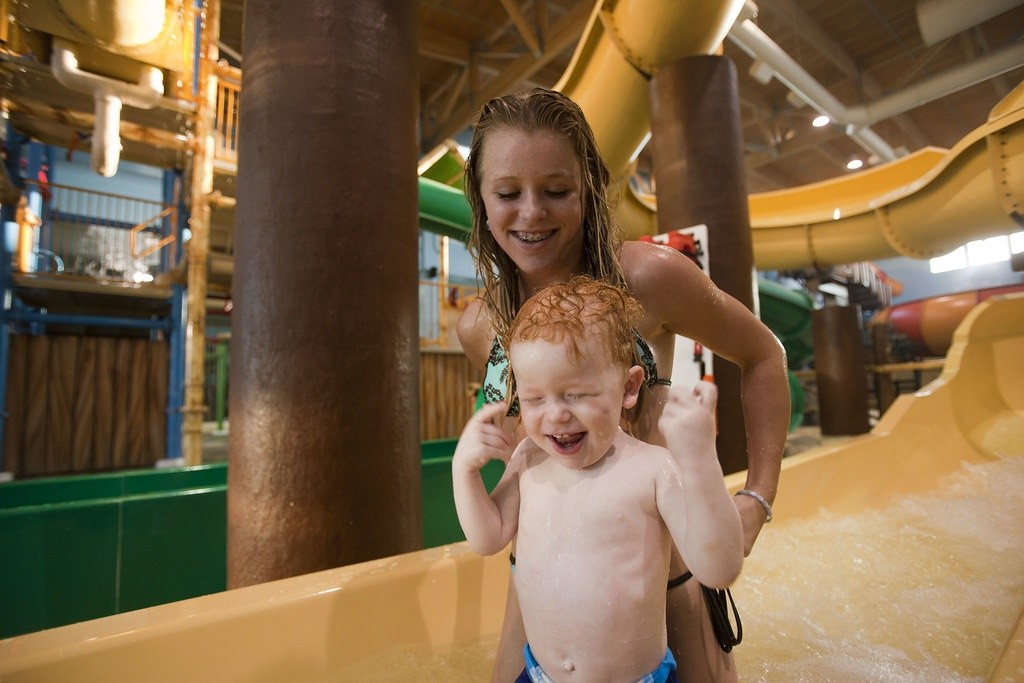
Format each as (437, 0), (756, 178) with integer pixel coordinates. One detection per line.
(732, 487), (774, 527)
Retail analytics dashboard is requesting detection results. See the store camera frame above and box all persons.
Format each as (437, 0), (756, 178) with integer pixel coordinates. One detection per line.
(455, 88), (793, 683)
(449, 275), (744, 683)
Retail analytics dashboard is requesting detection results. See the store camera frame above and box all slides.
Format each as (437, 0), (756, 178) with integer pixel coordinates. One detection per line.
(0, 0), (1024, 683)
